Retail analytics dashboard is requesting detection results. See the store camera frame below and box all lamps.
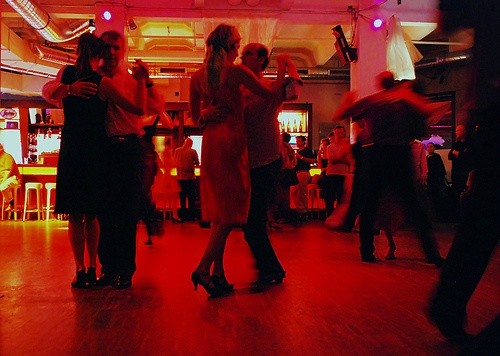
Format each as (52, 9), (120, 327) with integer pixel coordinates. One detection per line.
(128, 18), (137, 30)
(88, 19), (95, 31)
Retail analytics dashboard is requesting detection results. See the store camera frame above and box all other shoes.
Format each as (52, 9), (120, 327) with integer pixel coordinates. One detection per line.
(96, 271), (131, 289)
(71, 265), (97, 289)
(384, 244), (396, 260)
(251, 262), (286, 286)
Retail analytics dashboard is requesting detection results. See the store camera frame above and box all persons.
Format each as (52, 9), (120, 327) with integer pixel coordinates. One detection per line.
(0, 125), (480, 246)
(41, 30), (165, 289)
(333, 70), (446, 266)
(425, 0), (499, 348)
(187, 22), (304, 298)
(56, 35), (147, 288)
(199, 43), (287, 295)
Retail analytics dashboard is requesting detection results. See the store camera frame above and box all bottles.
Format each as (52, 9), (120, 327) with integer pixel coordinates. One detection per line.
(282, 121), (285, 133)
(287, 120), (291, 132)
(293, 119), (297, 132)
(47, 128), (52, 138)
(299, 121), (302, 132)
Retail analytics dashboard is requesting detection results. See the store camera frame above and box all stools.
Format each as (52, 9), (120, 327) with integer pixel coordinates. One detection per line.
(23, 182), (44, 222)
(2, 185), (23, 221)
(43, 183), (61, 220)
(311, 189), (328, 220)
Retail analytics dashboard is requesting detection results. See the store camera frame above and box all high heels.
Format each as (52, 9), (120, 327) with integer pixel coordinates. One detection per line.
(191, 270), (233, 295)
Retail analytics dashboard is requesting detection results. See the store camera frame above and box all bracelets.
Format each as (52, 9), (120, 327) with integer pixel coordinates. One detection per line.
(145, 81), (154, 88)
(66, 83), (72, 96)
